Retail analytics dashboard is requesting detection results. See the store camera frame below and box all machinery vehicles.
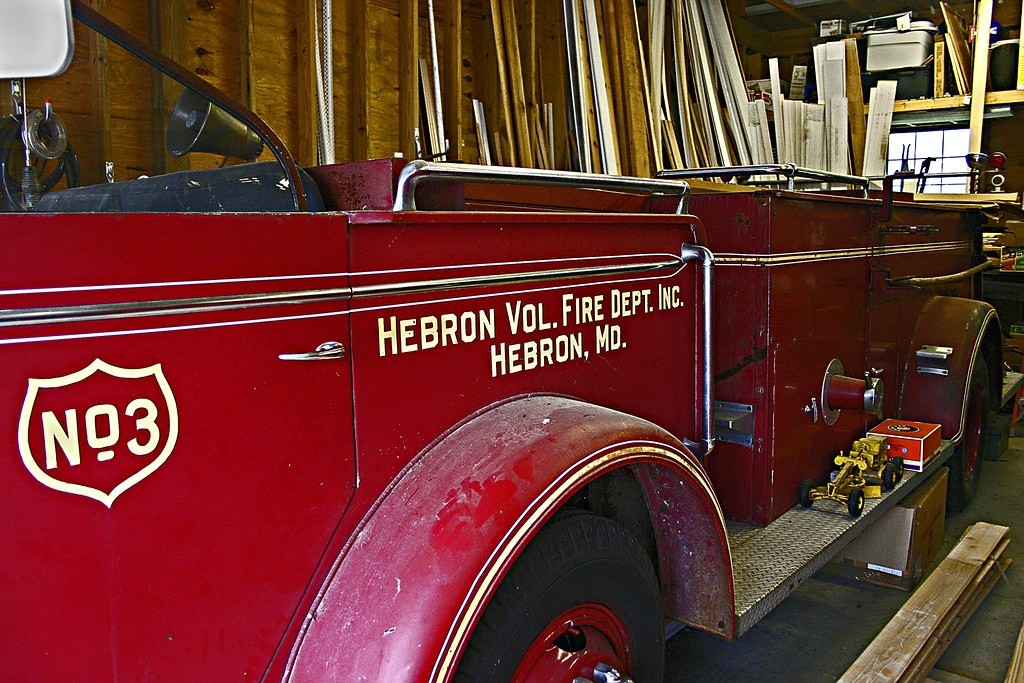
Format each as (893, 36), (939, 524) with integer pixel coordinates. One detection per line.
(798, 434), (907, 516)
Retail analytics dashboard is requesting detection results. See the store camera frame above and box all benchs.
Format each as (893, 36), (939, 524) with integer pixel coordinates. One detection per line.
(26, 159), (328, 211)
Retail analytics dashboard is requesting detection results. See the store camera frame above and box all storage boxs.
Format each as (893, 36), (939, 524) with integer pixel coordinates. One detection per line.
(860, 64), (933, 103)
(747, 66), (807, 112)
(865, 418), (941, 471)
(815, 466), (950, 593)
(865, 31), (934, 69)
(981, 212), (1024, 247)
(819, 19), (848, 37)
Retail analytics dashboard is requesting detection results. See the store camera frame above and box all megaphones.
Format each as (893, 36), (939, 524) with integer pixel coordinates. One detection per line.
(166, 88), (264, 161)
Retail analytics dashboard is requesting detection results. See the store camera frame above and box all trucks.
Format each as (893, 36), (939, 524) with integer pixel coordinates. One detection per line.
(0, 0), (1024, 682)
